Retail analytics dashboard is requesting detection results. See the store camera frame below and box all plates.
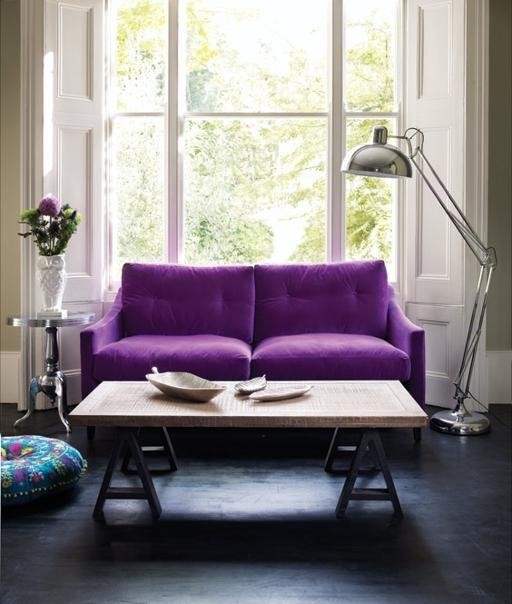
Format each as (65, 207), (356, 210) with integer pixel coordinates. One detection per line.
(144, 366), (228, 403)
(248, 382), (314, 401)
(233, 373), (267, 394)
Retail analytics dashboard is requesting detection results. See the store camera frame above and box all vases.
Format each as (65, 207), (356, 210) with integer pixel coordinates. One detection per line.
(38, 254), (67, 319)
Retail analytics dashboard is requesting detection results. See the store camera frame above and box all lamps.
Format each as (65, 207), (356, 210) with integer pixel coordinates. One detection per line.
(339, 125), (497, 436)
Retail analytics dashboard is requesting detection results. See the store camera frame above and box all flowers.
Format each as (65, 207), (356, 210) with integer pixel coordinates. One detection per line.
(17, 193), (82, 255)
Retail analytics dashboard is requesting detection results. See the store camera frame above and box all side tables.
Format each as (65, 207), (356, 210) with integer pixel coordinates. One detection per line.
(6, 311), (95, 432)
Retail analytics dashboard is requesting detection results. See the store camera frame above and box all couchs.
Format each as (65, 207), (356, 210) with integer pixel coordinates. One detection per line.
(80, 261), (424, 446)
